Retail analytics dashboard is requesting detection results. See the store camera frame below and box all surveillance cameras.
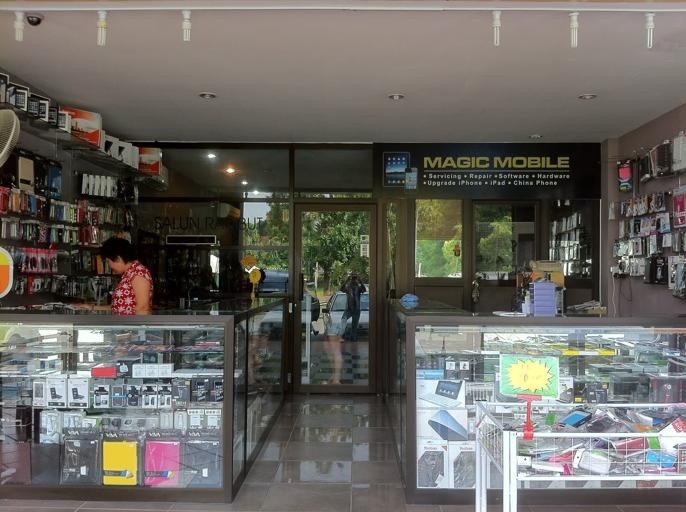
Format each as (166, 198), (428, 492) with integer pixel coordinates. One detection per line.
(24, 14), (45, 27)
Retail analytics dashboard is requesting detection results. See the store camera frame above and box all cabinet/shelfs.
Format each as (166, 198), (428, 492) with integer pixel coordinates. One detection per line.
(0, 296), (289, 504)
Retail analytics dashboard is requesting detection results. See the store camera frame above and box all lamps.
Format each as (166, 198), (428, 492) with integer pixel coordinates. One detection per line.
(15, 11), (25, 43)
(568, 12), (579, 49)
(491, 11), (503, 47)
(181, 10), (193, 43)
(645, 14), (656, 50)
(97, 12), (108, 48)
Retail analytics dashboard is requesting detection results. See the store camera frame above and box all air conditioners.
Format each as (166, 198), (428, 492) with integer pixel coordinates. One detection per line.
(216, 202), (240, 220)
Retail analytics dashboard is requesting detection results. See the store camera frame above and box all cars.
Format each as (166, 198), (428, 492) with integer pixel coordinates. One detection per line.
(322, 291), (370, 337)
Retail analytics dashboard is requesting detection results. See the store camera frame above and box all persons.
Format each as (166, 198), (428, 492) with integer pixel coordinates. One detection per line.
(321, 287), (348, 385)
(75, 237), (155, 315)
(302, 279), (320, 336)
(336, 272), (366, 342)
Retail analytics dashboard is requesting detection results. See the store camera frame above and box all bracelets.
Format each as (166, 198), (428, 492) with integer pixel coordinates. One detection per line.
(91, 304), (95, 312)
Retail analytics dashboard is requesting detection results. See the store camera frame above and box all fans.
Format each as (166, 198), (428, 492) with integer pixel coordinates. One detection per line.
(1, 108), (20, 169)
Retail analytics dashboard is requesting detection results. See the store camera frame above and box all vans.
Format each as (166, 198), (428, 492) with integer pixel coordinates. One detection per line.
(252, 269), (320, 323)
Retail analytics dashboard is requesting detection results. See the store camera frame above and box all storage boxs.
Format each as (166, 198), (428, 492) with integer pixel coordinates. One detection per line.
(0, 72), (163, 177)
